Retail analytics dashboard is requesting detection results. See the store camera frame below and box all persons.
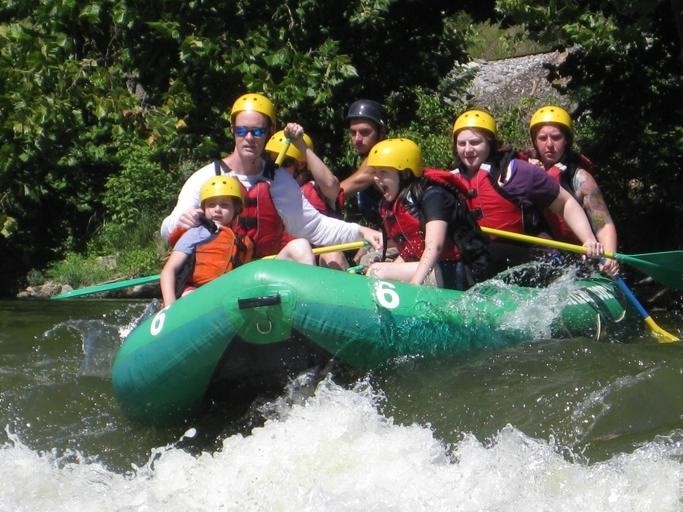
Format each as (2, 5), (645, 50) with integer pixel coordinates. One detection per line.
(161, 93), (384, 265)
(338, 100), (389, 266)
(160, 176), (318, 306)
(264, 121), (349, 270)
(517, 105), (622, 277)
(366, 137), (490, 290)
(449, 109), (604, 278)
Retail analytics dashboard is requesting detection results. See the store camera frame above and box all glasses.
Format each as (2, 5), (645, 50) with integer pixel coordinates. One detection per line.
(236, 126), (268, 137)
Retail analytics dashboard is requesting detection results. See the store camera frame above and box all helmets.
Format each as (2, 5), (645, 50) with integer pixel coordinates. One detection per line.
(199, 174), (246, 216)
(264, 130), (314, 163)
(529, 107), (574, 135)
(230, 93), (278, 129)
(453, 109), (497, 135)
(367, 137), (423, 177)
(348, 100), (388, 130)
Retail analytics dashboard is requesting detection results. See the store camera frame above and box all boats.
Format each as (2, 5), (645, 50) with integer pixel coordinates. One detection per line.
(110, 260), (634, 431)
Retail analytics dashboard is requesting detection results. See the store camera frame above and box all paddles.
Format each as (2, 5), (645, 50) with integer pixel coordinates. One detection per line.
(51, 239), (370, 300)
(600, 256), (680, 344)
(479, 224), (683, 291)
